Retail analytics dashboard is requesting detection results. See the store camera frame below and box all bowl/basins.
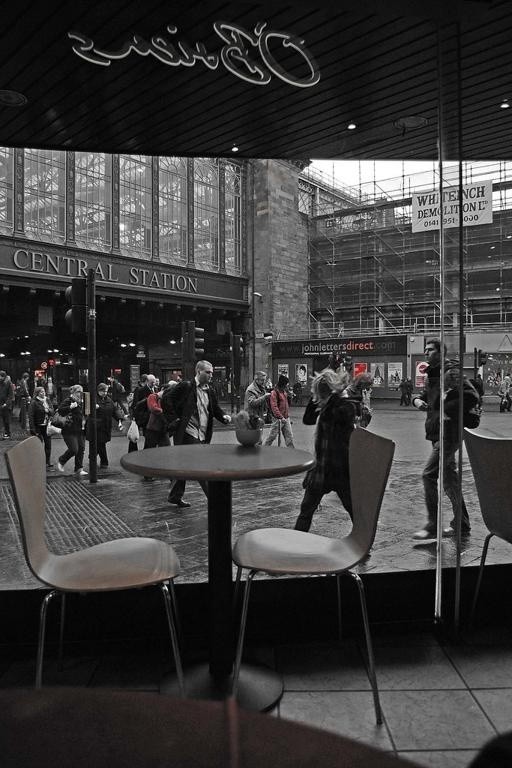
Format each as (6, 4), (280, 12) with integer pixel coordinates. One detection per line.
(234, 429), (262, 447)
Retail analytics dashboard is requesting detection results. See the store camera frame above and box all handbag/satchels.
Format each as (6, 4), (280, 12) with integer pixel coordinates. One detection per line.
(52, 409), (67, 429)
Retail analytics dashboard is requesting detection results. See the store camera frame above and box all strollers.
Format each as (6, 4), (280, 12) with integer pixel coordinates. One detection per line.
(290, 382), (303, 404)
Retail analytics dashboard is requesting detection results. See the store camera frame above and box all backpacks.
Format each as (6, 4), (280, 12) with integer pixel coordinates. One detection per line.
(443, 366), (484, 429)
(267, 388), (291, 414)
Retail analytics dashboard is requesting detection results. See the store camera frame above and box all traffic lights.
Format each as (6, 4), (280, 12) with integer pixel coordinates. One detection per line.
(241, 337), (244, 356)
(195, 327), (204, 355)
(481, 351), (487, 364)
(65, 285), (74, 329)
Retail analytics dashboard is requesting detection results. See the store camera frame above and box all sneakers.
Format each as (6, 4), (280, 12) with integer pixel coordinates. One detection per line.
(57, 462), (65, 472)
(3, 434), (10, 439)
(77, 470), (89, 475)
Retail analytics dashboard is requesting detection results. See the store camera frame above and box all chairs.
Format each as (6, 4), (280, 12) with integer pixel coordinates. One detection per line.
(462, 424), (512, 635)
(229, 425), (397, 726)
(2, 433), (187, 702)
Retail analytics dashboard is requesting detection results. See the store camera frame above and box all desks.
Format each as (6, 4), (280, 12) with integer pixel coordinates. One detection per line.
(120, 442), (319, 713)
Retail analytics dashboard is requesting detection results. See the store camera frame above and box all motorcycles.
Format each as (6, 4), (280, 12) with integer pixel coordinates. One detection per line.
(498, 373), (512, 412)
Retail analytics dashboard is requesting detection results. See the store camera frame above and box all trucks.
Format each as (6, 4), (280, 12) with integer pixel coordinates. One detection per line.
(50, 362), (74, 403)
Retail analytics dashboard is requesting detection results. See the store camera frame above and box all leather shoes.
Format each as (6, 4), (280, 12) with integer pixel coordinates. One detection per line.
(168, 497), (191, 508)
(443, 526), (471, 537)
(411, 529), (440, 541)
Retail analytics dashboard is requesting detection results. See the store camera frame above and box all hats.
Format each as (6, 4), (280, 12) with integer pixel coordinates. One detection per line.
(279, 375), (291, 385)
